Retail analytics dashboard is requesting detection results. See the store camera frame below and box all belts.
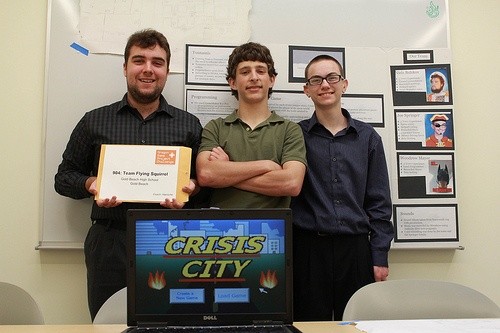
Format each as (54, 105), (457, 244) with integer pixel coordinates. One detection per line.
(91, 218), (128, 227)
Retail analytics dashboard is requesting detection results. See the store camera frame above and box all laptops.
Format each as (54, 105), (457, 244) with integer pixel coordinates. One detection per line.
(121, 208), (303, 333)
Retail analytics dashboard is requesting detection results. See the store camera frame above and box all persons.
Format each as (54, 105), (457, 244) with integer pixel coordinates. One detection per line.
(195, 42), (306, 209)
(287, 54), (394, 323)
(432, 164), (453, 193)
(426, 71), (450, 103)
(425, 114), (453, 148)
(53, 28), (203, 325)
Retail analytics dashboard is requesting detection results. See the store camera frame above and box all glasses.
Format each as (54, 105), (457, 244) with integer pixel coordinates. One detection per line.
(306, 72), (345, 86)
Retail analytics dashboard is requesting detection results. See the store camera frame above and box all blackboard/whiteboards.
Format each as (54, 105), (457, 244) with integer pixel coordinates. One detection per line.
(36, 1), (464, 250)
